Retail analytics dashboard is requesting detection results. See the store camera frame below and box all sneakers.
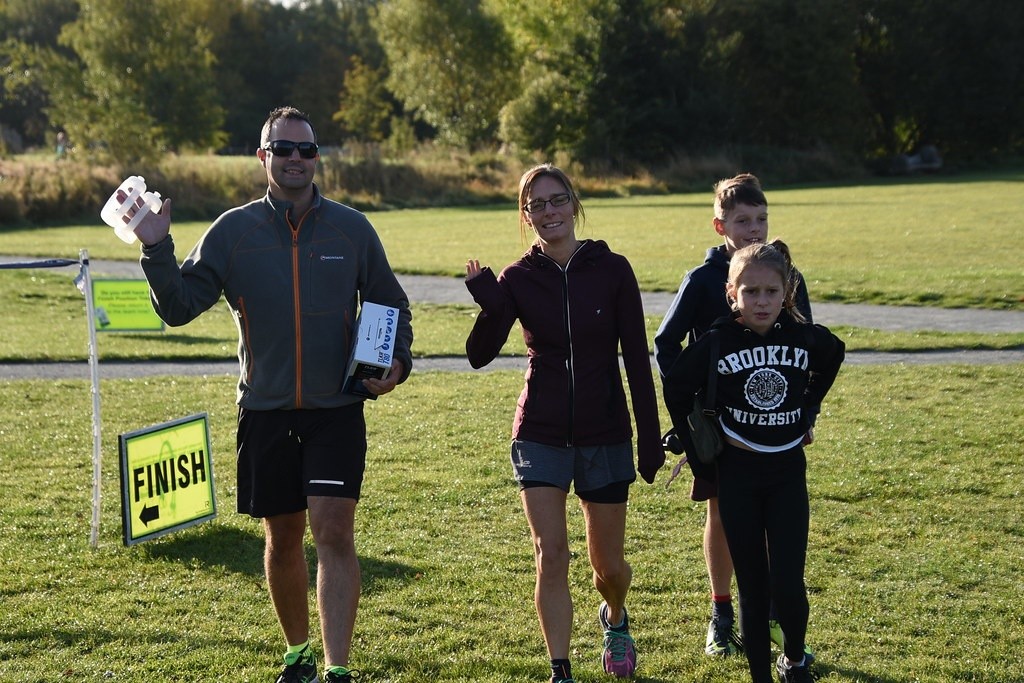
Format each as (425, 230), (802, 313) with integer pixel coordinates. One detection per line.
(324, 667), (360, 683)
(550, 677), (575, 683)
(776, 653), (820, 683)
(598, 600), (637, 677)
(274, 651), (320, 683)
(706, 614), (737, 657)
(769, 620), (812, 655)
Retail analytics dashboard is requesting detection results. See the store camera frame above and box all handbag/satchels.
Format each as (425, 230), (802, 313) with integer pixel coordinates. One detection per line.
(688, 330), (724, 464)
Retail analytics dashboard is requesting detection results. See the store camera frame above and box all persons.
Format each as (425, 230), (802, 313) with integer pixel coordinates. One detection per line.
(663, 238), (846, 683)
(463, 163), (665, 683)
(116, 107), (414, 683)
(654, 174), (813, 654)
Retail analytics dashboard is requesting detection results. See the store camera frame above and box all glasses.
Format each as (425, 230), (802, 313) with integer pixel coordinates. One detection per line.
(264, 140), (319, 159)
(524, 193), (571, 214)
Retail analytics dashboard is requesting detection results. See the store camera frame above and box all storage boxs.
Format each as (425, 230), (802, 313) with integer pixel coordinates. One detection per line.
(341, 301), (400, 400)
